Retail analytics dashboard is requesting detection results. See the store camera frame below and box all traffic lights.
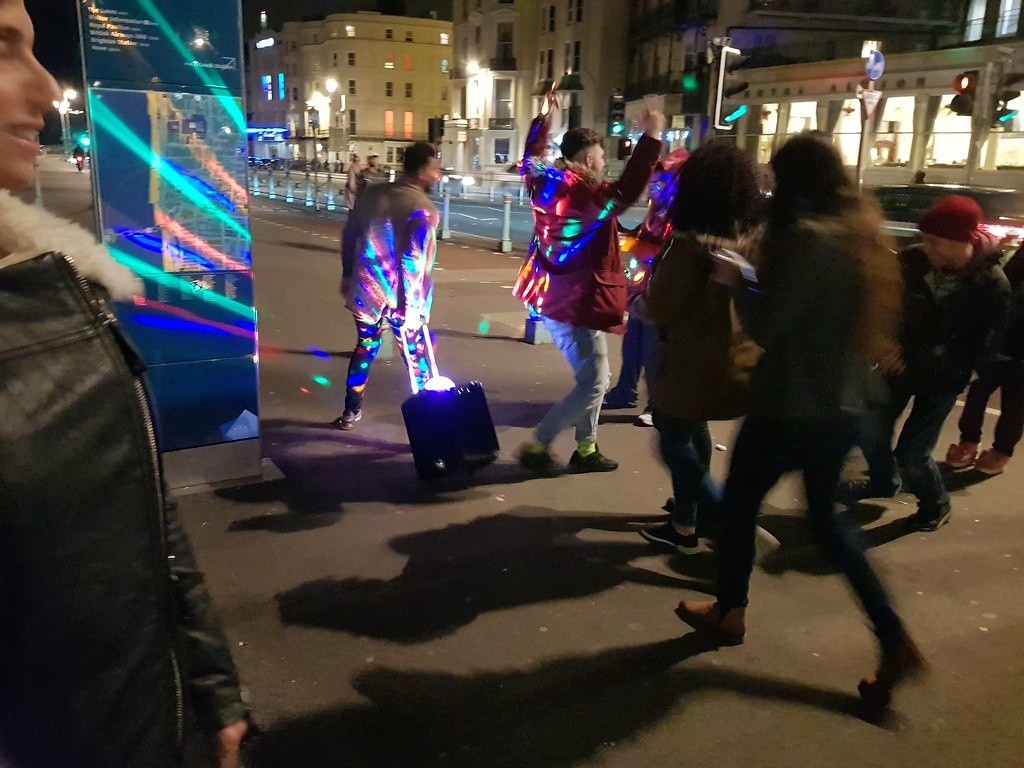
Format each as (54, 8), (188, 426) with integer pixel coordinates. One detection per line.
(680, 52), (711, 114)
(619, 139), (631, 160)
(607, 94), (626, 138)
(949, 69), (978, 116)
(991, 90), (1020, 125)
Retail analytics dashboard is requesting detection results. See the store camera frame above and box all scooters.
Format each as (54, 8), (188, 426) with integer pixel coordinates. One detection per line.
(75, 155), (84, 171)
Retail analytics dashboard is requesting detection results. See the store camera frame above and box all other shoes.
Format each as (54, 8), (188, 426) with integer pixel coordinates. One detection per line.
(858, 642), (925, 711)
(945, 440), (980, 467)
(601, 396), (619, 408)
(677, 596), (746, 642)
(842, 478), (878, 501)
(913, 499), (951, 531)
(516, 440), (563, 478)
(976, 448), (1012, 475)
(337, 408), (362, 430)
(640, 523), (698, 549)
(566, 442), (619, 474)
(636, 413), (654, 426)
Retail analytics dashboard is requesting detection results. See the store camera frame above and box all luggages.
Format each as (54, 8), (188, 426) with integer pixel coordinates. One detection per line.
(399, 324), (501, 481)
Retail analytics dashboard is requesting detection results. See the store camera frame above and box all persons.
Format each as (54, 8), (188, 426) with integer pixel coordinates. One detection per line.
(345, 154), (391, 214)
(638, 139), (779, 566)
(832, 197), (1024, 532)
(73, 144), (85, 167)
(674, 135), (927, 708)
(336, 142), (441, 430)
(0, 1), (248, 768)
(87, 145), (91, 165)
(513, 92), (692, 480)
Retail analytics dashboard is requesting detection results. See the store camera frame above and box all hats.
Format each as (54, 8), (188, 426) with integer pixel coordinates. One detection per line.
(917, 195), (982, 244)
(367, 152), (378, 158)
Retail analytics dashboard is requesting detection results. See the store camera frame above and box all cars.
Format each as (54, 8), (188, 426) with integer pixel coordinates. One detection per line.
(433, 167), (475, 199)
